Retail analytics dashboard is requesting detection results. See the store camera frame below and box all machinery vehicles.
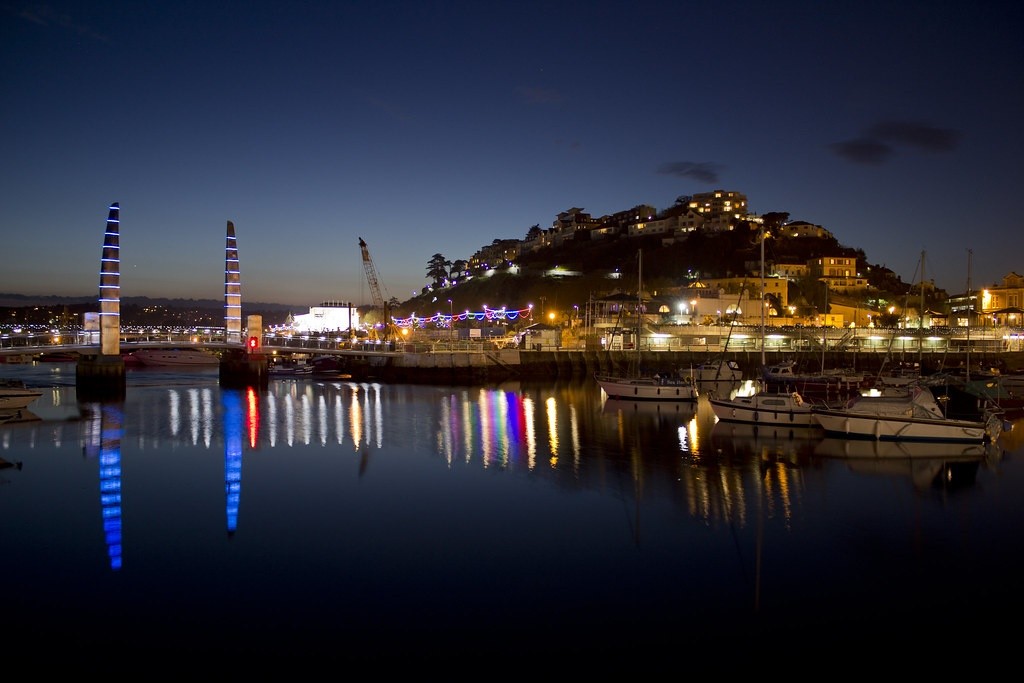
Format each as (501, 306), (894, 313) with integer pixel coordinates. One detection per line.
(358, 236), (384, 310)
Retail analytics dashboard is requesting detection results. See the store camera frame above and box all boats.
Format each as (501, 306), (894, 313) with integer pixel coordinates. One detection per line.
(271, 361), (311, 375)
(676, 359), (744, 383)
(765, 363), (1024, 406)
(0, 378), (45, 409)
(310, 368), (353, 381)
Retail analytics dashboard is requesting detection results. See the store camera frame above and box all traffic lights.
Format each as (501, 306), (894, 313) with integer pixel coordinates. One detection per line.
(250, 337), (258, 348)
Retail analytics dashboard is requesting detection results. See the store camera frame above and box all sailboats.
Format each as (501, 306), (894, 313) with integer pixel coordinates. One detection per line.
(920, 248), (1015, 400)
(810, 249), (985, 443)
(708, 224), (843, 428)
(594, 249), (700, 402)
